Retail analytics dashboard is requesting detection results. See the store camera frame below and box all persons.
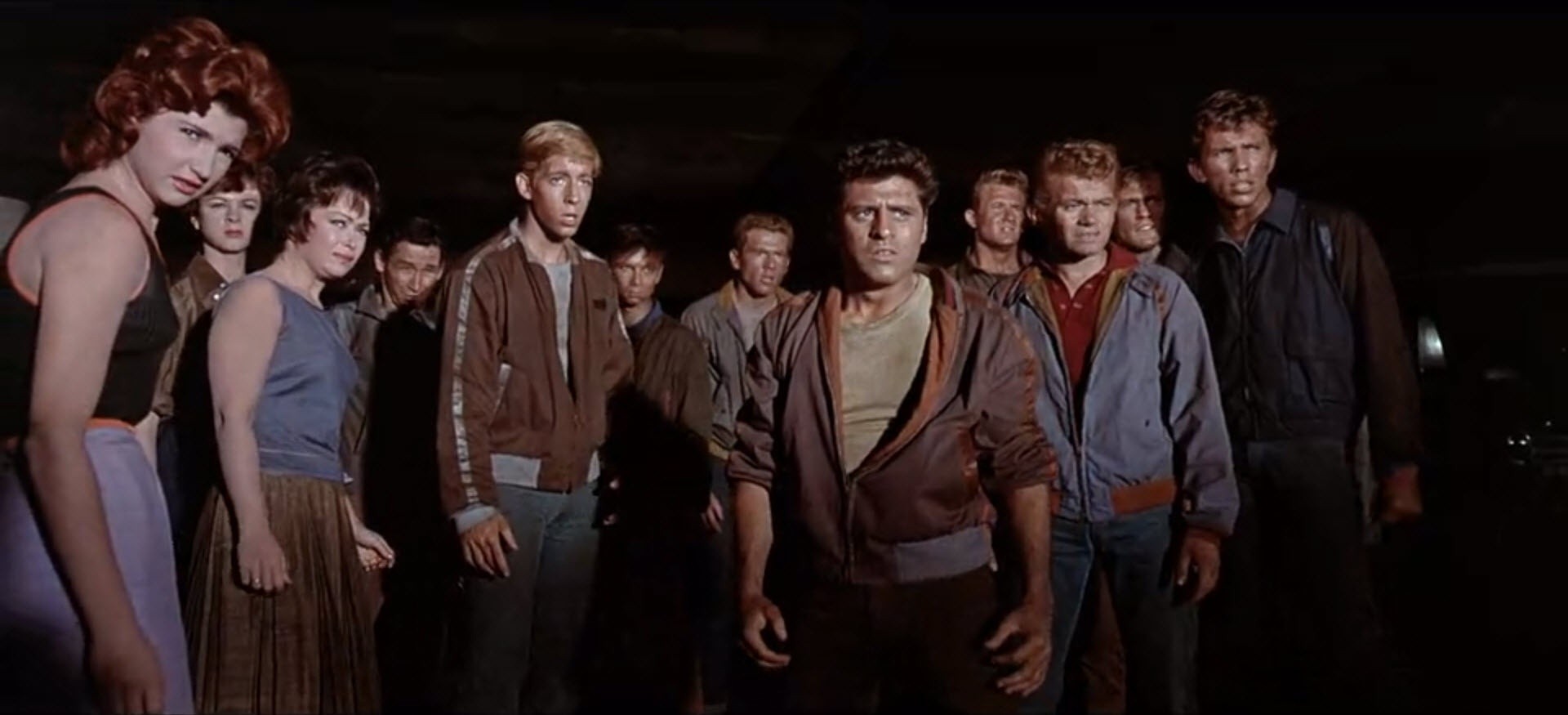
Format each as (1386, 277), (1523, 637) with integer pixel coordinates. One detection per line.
(946, 164), (1034, 297)
(1181, 90), (1424, 715)
(155, 152), (284, 600)
(722, 137), (1060, 715)
(186, 151), (395, 715)
(575, 215), (709, 715)
(993, 140), (1242, 715)
(0, 15), (292, 715)
(681, 213), (806, 715)
(1069, 162), (1195, 715)
(429, 120), (637, 715)
(333, 218), (452, 715)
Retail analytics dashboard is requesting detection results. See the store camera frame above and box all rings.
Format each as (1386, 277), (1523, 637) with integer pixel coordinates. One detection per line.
(252, 579), (261, 584)
